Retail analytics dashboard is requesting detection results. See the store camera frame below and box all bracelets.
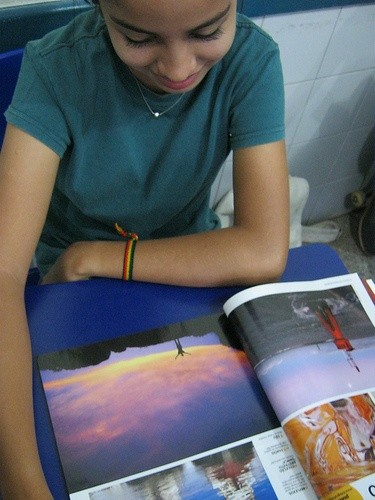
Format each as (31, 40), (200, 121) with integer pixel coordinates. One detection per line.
(115, 223), (138, 281)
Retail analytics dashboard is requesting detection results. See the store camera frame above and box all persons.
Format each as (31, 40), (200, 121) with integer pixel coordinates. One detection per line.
(0, 0), (290, 500)
(174, 338), (190, 359)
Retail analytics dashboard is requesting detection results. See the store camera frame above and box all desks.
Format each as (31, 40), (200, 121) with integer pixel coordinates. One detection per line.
(23, 243), (352, 500)
